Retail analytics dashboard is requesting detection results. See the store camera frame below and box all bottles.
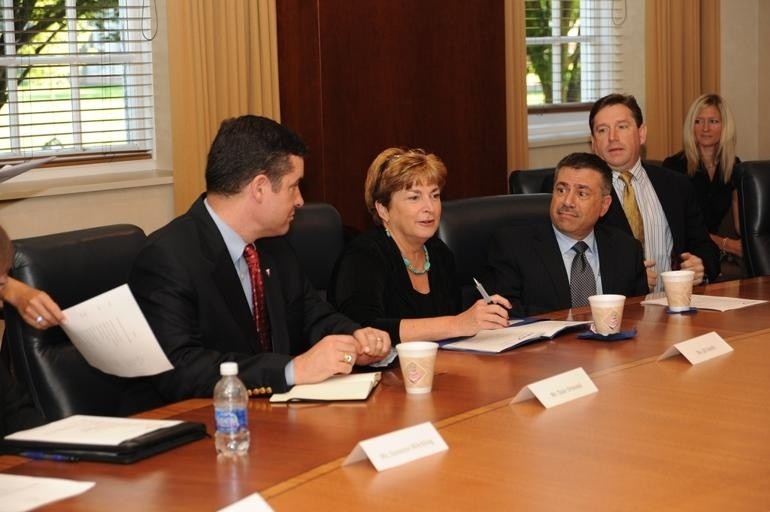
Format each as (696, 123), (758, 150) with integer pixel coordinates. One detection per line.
(212, 362), (250, 456)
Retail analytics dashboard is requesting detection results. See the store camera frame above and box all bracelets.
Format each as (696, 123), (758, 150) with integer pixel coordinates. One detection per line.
(721, 237), (729, 254)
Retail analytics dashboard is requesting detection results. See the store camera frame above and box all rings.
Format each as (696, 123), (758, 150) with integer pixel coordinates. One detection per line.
(36, 315), (44, 324)
(376, 335), (384, 343)
(342, 354), (354, 365)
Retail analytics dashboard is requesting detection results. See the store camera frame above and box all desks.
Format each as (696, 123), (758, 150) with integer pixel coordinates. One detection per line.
(1, 278), (770, 512)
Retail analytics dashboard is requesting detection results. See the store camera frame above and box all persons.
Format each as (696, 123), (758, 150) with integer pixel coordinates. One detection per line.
(322, 144), (516, 348)
(475, 151), (649, 324)
(660, 92), (744, 264)
(2, 275), (67, 335)
(95, 112), (393, 420)
(0, 227), (18, 354)
(581, 93), (723, 299)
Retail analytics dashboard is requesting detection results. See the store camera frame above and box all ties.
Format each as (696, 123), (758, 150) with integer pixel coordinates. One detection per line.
(565, 238), (600, 309)
(240, 242), (275, 355)
(615, 172), (652, 264)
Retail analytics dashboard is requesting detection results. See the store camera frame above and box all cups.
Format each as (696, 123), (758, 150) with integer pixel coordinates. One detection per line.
(396, 341), (439, 395)
(659, 270), (695, 311)
(587, 294), (626, 336)
(663, 315), (693, 352)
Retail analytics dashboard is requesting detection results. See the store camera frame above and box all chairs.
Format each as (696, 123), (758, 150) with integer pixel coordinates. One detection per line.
(3, 224), (167, 425)
(734, 161), (770, 277)
(433, 168), (557, 313)
(289, 202), (343, 304)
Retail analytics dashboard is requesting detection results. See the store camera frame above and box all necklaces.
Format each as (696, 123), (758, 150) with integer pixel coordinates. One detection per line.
(395, 241), (432, 278)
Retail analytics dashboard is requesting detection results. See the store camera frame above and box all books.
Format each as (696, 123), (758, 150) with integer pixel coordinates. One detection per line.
(5, 412), (209, 465)
(265, 369), (384, 406)
(440, 313), (597, 360)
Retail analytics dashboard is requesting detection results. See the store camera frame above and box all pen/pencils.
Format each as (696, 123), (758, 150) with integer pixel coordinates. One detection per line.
(472, 276), (493, 305)
(19, 451), (81, 462)
